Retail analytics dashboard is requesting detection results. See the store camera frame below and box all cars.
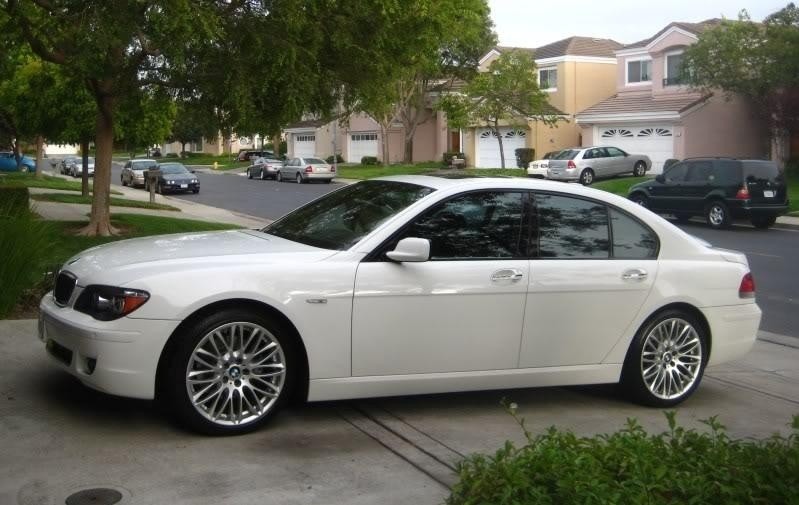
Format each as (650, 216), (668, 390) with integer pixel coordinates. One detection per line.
(0, 150), (36, 173)
(545, 145), (653, 186)
(35, 169), (768, 441)
(526, 150), (562, 178)
(236, 148), (337, 185)
(52, 156), (202, 196)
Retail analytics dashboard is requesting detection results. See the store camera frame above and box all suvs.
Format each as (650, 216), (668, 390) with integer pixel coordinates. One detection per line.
(626, 153), (792, 229)
